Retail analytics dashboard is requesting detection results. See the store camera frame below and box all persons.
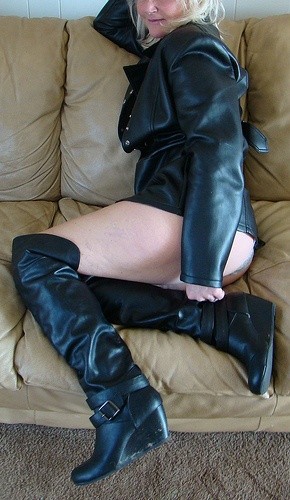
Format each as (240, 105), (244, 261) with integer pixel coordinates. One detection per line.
(9, 1), (279, 488)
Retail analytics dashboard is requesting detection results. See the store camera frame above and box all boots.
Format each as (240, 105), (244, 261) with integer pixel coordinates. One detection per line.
(76, 273), (276, 394)
(12, 234), (169, 485)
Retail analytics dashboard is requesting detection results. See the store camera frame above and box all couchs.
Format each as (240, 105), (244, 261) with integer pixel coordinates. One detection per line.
(2, 14), (289, 434)
